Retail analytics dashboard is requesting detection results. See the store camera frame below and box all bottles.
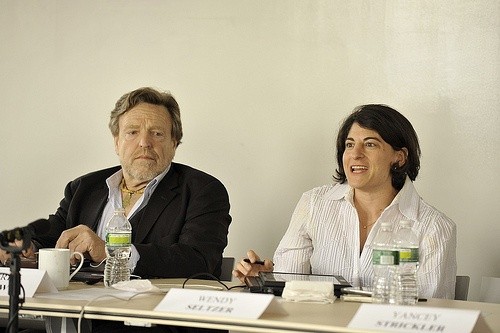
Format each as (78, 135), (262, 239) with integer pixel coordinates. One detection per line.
(103, 207), (132, 286)
(395, 220), (420, 305)
(372, 222), (399, 305)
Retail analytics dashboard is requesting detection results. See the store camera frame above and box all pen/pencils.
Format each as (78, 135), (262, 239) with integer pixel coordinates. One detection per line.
(243, 258), (276, 266)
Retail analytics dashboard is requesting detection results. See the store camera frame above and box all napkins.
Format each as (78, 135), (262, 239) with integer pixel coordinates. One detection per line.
(282, 282), (336, 304)
(111, 279), (161, 293)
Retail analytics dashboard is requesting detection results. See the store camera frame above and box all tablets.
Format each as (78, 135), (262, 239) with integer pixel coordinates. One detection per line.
(258, 271), (351, 289)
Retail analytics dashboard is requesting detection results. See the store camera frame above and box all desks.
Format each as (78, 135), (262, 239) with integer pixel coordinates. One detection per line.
(0, 277), (500, 333)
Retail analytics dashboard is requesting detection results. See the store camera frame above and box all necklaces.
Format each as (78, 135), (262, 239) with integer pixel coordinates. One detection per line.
(360, 221), (376, 229)
(121, 178), (144, 209)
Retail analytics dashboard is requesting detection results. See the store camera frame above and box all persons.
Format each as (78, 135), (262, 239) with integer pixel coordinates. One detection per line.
(0, 87), (232, 333)
(233, 104), (457, 299)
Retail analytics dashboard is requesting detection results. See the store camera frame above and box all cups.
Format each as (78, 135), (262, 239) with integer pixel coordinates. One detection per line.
(38, 249), (83, 288)
